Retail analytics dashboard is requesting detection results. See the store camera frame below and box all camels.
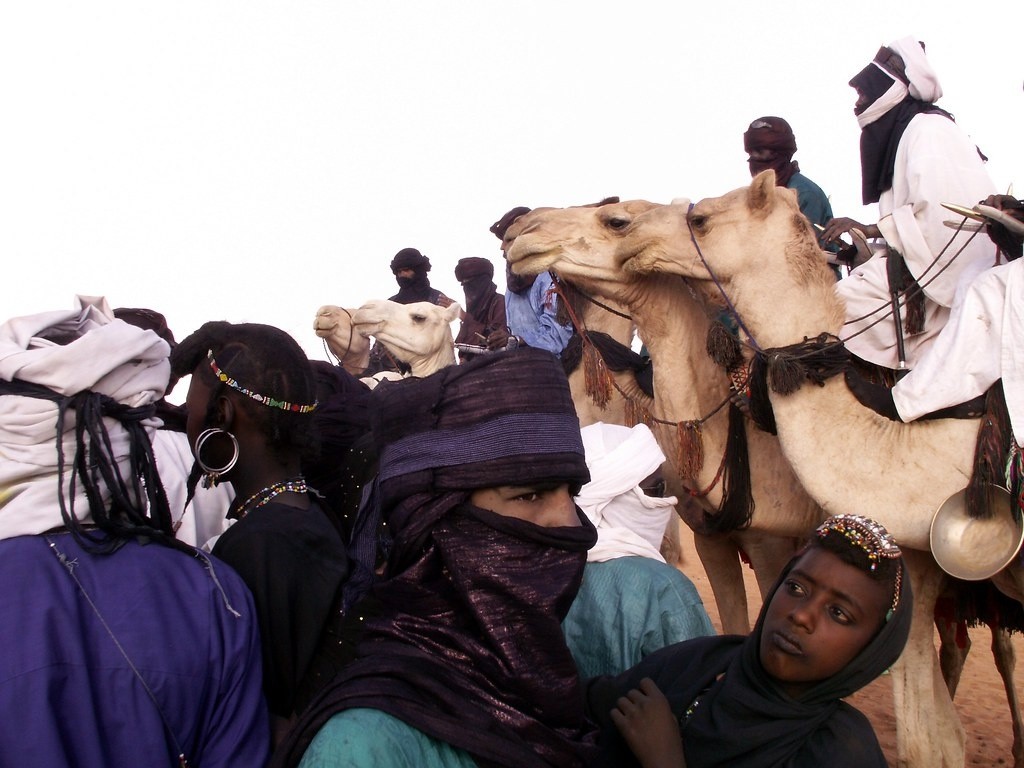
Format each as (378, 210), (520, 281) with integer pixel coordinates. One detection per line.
(316, 301), (460, 391)
(500, 168), (1024, 768)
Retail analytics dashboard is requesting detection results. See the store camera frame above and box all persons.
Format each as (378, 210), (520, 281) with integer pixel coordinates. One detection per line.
(183, 323), (352, 745)
(731, 116), (844, 419)
(303, 347), (719, 767)
(487, 207), (575, 358)
(0, 295), (267, 768)
(369, 249), (446, 375)
(820, 39), (1007, 374)
(450, 253), (507, 365)
(610, 515), (914, 768)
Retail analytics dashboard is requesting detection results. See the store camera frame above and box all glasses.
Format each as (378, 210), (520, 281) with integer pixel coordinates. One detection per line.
(751, 120), (772, 129)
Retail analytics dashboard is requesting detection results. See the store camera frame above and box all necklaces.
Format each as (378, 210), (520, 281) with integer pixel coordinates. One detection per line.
(236, 475), (309, 517)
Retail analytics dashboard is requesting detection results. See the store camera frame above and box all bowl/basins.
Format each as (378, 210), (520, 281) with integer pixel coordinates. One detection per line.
(930, 482), (1024, 581)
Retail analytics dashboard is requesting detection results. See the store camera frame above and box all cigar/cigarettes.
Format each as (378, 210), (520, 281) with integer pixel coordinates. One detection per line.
(474, 332), (487, 340)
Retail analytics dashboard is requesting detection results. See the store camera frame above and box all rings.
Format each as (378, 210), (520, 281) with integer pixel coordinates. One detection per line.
(448, 300), (449, 302)
